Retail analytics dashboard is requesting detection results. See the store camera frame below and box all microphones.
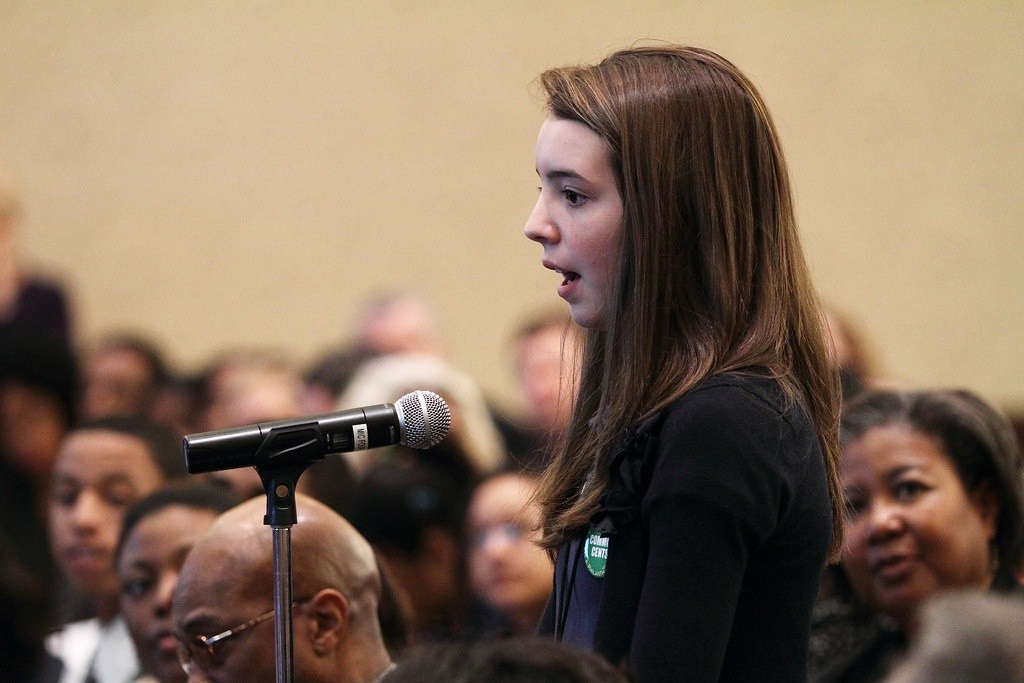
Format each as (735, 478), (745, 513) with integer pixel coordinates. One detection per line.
(184, 390), (452, 475)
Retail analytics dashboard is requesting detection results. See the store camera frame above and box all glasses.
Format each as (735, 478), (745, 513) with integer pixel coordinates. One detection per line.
(176, 596), (314, 676)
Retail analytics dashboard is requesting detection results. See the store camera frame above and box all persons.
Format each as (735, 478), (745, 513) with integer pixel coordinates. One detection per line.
(0, 194), (629, 683)
(807, 296), (1024, 683)
(521, 42), (847, 683)
